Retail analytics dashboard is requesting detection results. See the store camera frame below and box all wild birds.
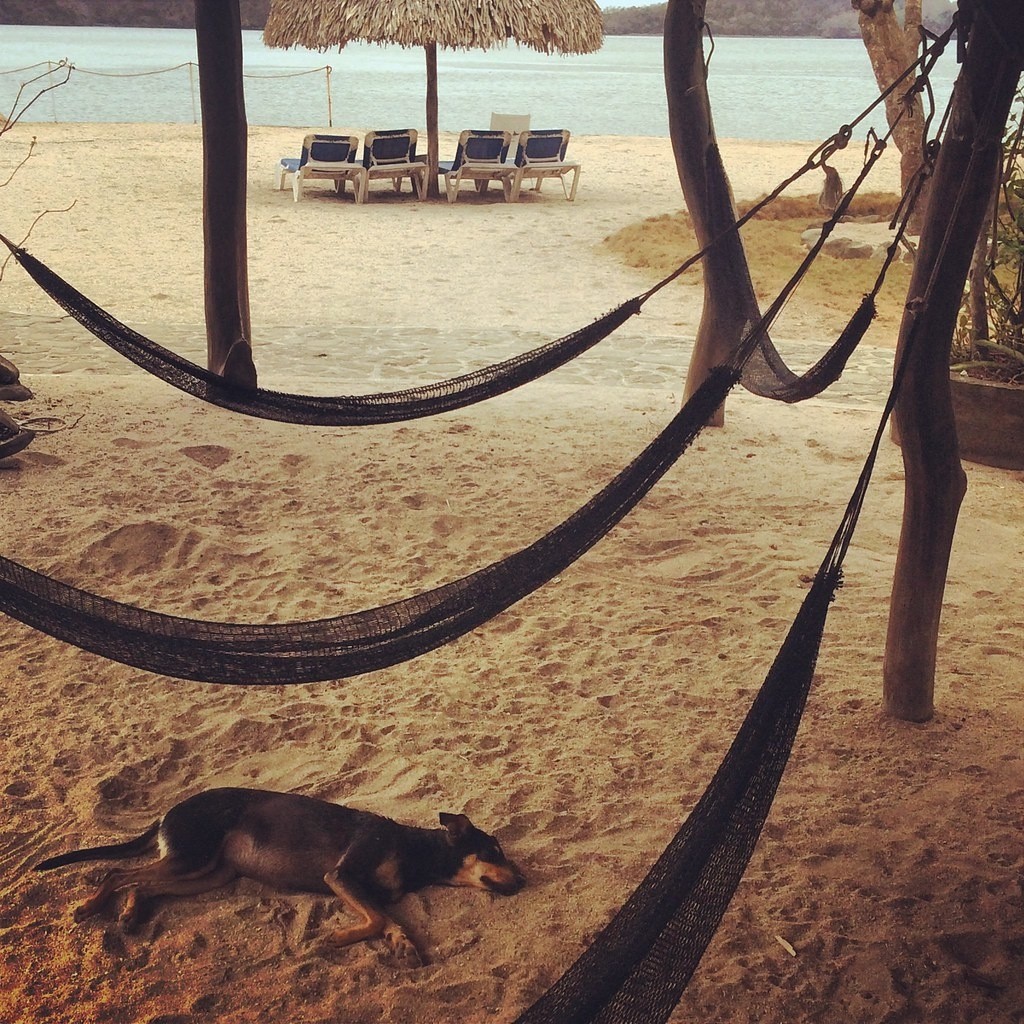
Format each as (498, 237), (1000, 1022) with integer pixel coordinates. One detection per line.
(219, 337), (257, 388)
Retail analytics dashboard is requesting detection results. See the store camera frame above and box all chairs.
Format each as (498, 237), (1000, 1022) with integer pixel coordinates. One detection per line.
(277, 112), (584, 205)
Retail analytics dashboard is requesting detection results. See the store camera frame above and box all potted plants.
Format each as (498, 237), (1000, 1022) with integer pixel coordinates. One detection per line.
(885, 67), (1023, 473)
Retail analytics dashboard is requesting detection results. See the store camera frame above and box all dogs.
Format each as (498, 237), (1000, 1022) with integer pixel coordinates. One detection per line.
(32, 786), (527, 972)
